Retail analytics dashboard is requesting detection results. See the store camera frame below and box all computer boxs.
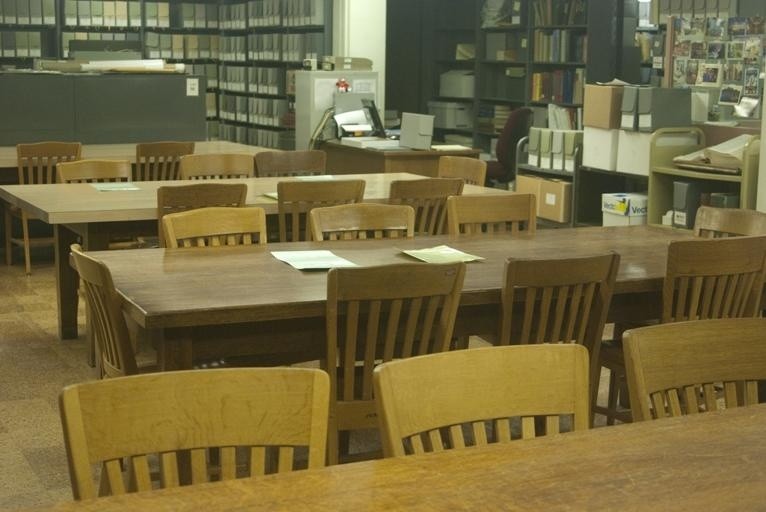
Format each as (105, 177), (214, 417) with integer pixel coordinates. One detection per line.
(332, 92), (375, 139)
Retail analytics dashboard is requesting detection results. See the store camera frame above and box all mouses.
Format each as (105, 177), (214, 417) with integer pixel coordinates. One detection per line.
(390, 135), (399, 140)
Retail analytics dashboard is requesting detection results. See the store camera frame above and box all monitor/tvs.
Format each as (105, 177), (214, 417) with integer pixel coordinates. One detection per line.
(361, 99), (387, 138)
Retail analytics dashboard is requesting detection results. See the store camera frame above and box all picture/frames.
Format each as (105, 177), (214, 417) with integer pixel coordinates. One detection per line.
(673, 41), (761, 106)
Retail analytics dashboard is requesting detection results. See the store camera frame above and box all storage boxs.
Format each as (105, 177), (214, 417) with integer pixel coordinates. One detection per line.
(515, 84), (691, 228)
(426, 69), (475, 148)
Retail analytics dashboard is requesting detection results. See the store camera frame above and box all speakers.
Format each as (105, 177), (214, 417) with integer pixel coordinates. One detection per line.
(302, 58), (317, 70)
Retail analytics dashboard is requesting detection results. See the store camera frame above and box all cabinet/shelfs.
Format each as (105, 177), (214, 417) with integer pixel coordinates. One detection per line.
(0, 1), (333, 150)
(422, 0), (621, 158)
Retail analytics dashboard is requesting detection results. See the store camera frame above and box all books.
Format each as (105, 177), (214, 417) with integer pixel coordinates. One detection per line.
(496, 49), (517, 62)
(494, 105), (510, 135)
(505, 66), (526, 79)
(531, 1), (587, 129)
(672, 134), (758, 169)
